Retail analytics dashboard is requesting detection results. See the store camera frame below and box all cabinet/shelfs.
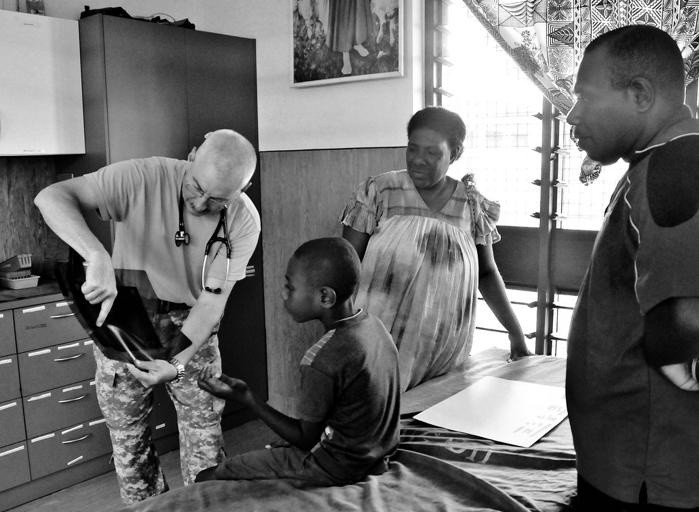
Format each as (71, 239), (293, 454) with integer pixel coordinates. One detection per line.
(1, 309), (33, 512)
(79, 14), (269, 458)
(0, 9), (87, 159)
(13, 300), (121, 502)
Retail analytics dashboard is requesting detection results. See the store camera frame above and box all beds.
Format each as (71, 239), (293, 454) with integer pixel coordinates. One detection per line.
(122, 347), (609, 512)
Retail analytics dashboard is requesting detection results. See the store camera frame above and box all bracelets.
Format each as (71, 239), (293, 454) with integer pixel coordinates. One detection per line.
(168, 357), (185, 384)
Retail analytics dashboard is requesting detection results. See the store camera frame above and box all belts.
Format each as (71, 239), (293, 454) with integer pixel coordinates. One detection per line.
(160, 300), (193, 312)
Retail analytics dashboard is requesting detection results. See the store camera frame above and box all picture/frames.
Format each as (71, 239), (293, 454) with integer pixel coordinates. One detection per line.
(290, 0), (404, 88)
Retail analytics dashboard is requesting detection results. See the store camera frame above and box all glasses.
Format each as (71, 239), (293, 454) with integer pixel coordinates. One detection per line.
(185, 166), (239, 208)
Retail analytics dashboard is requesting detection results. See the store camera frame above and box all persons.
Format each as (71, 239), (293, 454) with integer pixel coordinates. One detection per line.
(30, 127), (263, 508)
(190, 236), (405, 489)
(324, 0), (372, 75)
(562, 24), (698, 512)
(335, 105), (537, 396)
